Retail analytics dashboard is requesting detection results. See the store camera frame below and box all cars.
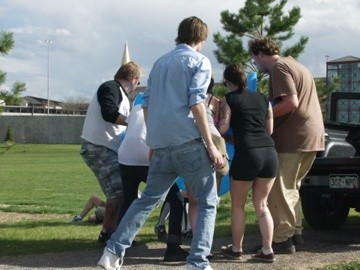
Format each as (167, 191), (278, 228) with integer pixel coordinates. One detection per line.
(299, 124), (360, 231)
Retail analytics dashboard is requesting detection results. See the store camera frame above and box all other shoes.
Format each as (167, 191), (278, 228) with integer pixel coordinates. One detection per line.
(207, 253), (214, 259)
(182, 229), (194, 239)
(291, 233), (305, 247)
(165, 249), (189, 264)
(155, 225), (168, 241)
(221, 243), (242, 260)
(252, 240), (296, 254)
(69, 215), (82, 222)
(96, 250), (122, 270)
(98, 231), (137, 246)
(253, 248), (276, 262)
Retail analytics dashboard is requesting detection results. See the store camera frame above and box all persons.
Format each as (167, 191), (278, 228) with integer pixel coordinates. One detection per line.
(218, 65), (280, 263)
(97, 16), (223, 269)
(81, 62), (139, 250)
(72, 190), (142, 222)
(249, 36), (325, 255)
(185, 78), (221, 259)
(115, 91), (190, 262)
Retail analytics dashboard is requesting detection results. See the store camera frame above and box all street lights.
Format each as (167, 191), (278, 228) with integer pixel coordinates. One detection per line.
(44, 39), (56, 115)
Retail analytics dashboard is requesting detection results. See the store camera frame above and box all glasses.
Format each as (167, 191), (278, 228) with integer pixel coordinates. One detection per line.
(223, 80), (228, 88)
(134, 77), (140, 85)
